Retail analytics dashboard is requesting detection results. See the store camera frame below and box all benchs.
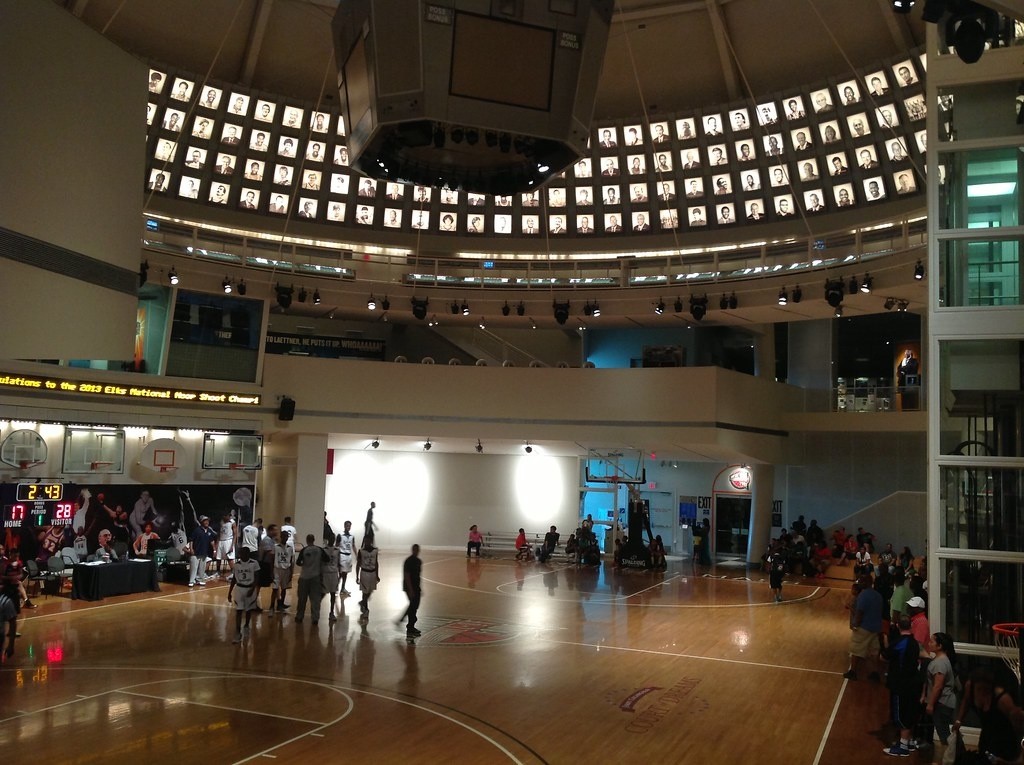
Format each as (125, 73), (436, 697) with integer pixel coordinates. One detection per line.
(465, 531), (576, 557)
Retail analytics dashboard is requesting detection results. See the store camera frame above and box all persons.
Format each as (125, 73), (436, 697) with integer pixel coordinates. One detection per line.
(94, 540), (118, 559)
(762, 514), (956, 757)
(951, 669), (1024, 765)
(398, 544), (421, 634)
(540, 526), (560, 563)
(647, 535), (667, 567)
(612, 535), (628, 561)
(182, 510), (380, 643)
(514, 528), (529, 560)
(696, 518), (710, 564)
(364, 501), (379, 535)
(0, 542), (36, 661)
(565, 514), (601, 564)
(168, 489), (200, 550)
(34, 490), (161, 560)
(466, 525), (484, 558)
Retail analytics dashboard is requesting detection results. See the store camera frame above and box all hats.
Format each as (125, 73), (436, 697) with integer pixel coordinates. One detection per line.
(199, 515), (210, 522)
(905, 596), (925, 609)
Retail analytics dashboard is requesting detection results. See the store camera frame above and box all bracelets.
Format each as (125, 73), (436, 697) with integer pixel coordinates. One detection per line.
(955, 720), (961, 725)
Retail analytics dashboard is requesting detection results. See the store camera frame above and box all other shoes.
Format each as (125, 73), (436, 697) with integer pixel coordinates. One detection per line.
(253, 603), (291, 617)
(867, 670), (880, 682)
(197, 581), (207, 586)
(773, 594), (784, 603)
(329, 612), (336, 620)
(226, 574), (233, 580)
(340, 589), (351, 596)
(311, 616), (318, 624)
(232, 633), (242, 643)
(189, 583), (194, 586)
(243, 625), (249, 637)
(843, 670), (857, 681)
(6, 630), (22, 638)
(883, 737), (934, 757)
(358, 601), (369, 615)
(25, 599), (37, 609)
(295, 617), (302, 623)
(407, 627), (421, 636)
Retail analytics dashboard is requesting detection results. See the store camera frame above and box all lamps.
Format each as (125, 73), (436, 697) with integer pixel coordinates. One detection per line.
(236, 279), (247, 296)
(479, 321), (486, 330)
(371, 437), (380, 449)
(429, 316), (438, 326)
(384, 315), (388, 321)
(167, 265), (179, 285)
(381, 297), (392, 310)
(450, 300), (459, 314)
(297, 286), (308, 303)
(366, 294), (376, 310)
(461, 302), (470, 316)
(474, 438), (484, 454)
(524, 440), (533, 453)
(330, 312), (334, 319)
(662, 461), (679, 469)
(221, 275), (232, 294)
(501, 262), (943, 329)
(423, 438), (432, 451)
(313, 289), (322, 306)
(278, 395), (296, 423)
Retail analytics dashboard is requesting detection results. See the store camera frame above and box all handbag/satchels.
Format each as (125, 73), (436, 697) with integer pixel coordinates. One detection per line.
(942, 730), (957, 765)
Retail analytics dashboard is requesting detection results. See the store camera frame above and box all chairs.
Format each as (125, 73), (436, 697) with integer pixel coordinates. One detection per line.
(27, 560), (58, 600)
(166, 548), (181, 564)
(47, 556), (72, 594)
(62, 547), (80, 573)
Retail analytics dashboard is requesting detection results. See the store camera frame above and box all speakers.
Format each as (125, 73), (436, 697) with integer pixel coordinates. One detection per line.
(278, 399), (296, 421)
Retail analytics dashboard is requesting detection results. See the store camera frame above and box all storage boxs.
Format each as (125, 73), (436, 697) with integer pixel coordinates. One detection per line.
(604, 538), (622, 553)
(605, 529), (623, 539)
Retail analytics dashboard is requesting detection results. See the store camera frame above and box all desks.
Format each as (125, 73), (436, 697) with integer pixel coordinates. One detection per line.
(71, 558), (161, 602)
